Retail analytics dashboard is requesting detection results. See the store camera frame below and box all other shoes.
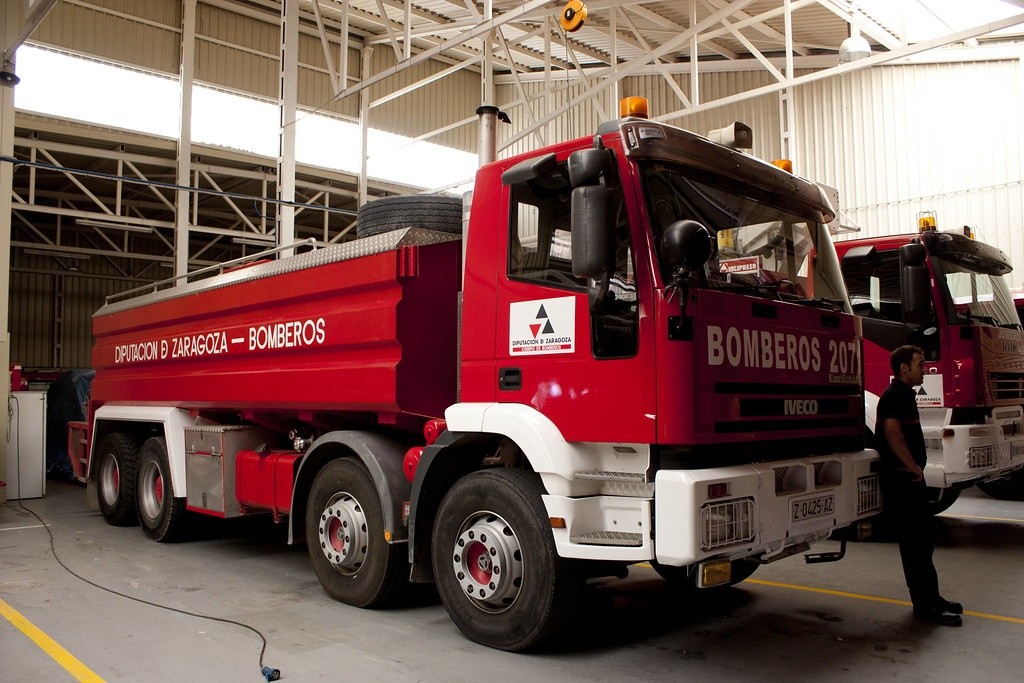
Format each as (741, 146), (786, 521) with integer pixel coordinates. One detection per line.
(914, 610), (962, 627)
(939, 598), (963, 613)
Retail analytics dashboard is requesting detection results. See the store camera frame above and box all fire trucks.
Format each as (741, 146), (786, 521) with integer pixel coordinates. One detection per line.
(719, 211), (1024, 513)
(85, 96), (885, 653)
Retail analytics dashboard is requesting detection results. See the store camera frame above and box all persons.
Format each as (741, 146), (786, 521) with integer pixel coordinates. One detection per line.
(874, 346), (965, 626)
